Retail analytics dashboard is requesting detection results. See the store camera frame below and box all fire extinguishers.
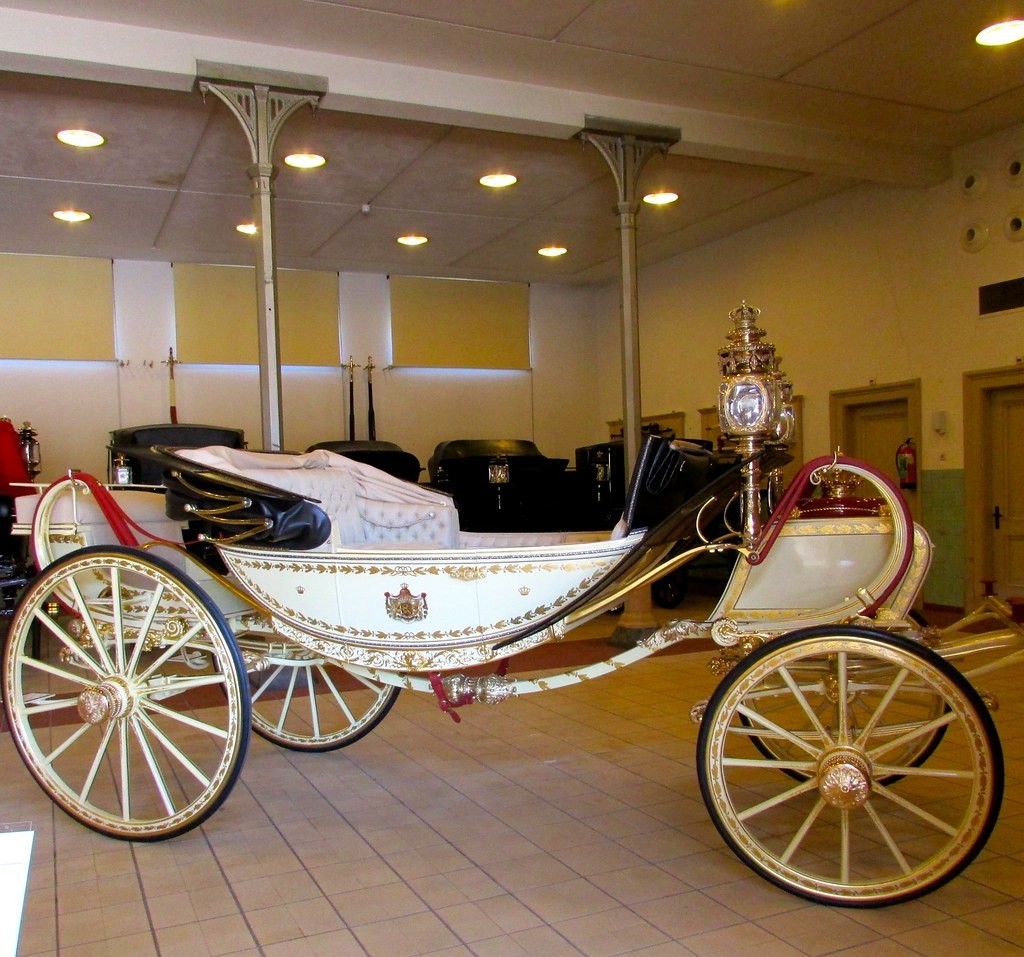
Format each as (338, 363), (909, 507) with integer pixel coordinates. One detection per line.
(896, 436), (918, 491)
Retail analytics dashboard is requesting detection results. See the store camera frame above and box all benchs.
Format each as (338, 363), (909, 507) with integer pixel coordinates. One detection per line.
(175, 448), (459, 550)
